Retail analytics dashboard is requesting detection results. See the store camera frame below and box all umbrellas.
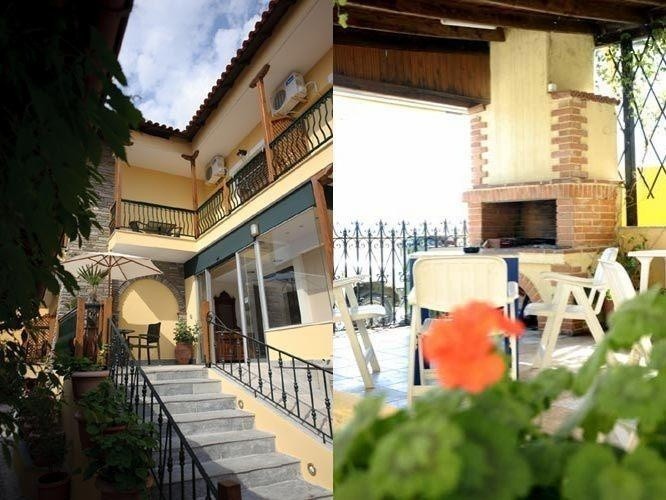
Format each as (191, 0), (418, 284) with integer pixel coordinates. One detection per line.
(55, 250), (164, 295)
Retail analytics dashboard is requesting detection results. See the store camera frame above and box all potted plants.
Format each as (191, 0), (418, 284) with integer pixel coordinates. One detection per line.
(77, 265), (109, 360)
(0, 340), (161, 500)
(172, 318), (199, 364)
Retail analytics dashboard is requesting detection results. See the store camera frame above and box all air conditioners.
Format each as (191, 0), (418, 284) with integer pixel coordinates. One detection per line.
(269, 72), (307, 118)
(205, 155), (226, 184)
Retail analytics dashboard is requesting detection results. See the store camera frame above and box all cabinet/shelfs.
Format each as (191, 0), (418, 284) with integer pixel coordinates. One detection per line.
(214, 332), (244, 363)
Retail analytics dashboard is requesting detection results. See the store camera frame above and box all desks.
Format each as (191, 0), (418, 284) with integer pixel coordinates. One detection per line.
(627, 249), (665, 292)
(120, 330), (135, 338)
(409, 249), (519, 385)
(143, 221), (176, 236)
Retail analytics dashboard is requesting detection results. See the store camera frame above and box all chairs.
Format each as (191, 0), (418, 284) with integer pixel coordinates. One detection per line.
(333, 276), (386, 388)
(129, 221), (148, 232)
(600, 249), (666, 359)
(524, 248), (618, 372)
(172, 227), (183, 236)
(128, 322), (161, 365)
(407, 256), (518, 408)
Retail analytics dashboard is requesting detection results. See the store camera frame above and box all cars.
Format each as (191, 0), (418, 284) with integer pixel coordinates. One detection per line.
(330, 250), (404, 334)
